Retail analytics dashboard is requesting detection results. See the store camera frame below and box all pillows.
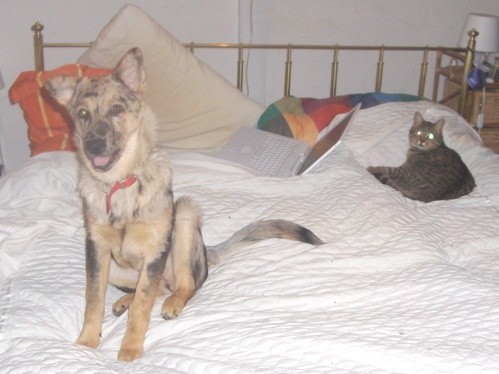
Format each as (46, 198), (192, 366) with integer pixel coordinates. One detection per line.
(76, 3), (266, 154)
(8, 63), (114, 158)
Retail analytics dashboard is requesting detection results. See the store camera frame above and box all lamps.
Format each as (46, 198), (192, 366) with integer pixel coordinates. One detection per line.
(457, 12), (499, 63)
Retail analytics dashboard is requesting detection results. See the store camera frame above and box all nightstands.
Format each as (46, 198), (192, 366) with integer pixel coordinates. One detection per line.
(438, 66), (499, 154)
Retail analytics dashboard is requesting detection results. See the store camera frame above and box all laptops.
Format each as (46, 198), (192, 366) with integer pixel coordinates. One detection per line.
(215, 103), (361, 178)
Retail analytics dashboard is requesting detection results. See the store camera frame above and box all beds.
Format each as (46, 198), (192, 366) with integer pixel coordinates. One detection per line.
(0, 21), (499, 374)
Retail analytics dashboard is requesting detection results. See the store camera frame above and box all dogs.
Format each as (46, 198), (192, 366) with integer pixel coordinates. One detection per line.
(44, 47), (325, 363)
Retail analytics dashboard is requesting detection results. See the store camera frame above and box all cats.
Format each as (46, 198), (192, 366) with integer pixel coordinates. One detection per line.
(366, 112), (477, 202)
